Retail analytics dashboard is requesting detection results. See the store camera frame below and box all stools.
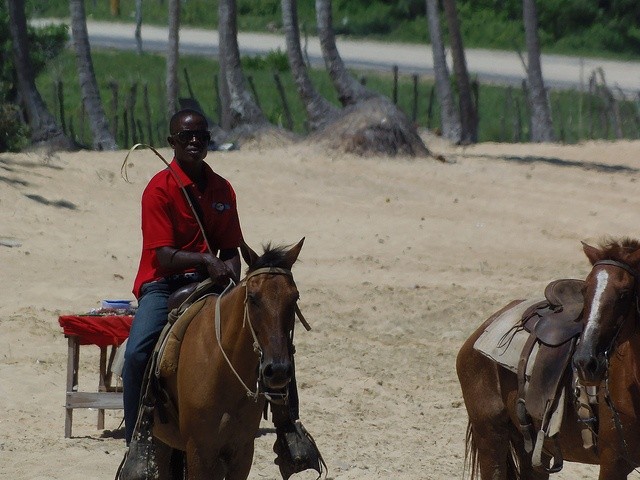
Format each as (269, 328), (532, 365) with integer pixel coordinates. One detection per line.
(58, 313), (135, 438)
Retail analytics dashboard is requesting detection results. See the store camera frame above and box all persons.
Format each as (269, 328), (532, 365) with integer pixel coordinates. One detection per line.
(123, 109), (244, 447)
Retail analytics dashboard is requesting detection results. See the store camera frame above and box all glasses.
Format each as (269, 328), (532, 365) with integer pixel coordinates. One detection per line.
(171, 130), (211, 139)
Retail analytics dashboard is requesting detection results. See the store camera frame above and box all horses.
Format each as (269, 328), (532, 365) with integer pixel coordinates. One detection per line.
(138, 235), (305, 480)
(456, 235), (640, 479)
